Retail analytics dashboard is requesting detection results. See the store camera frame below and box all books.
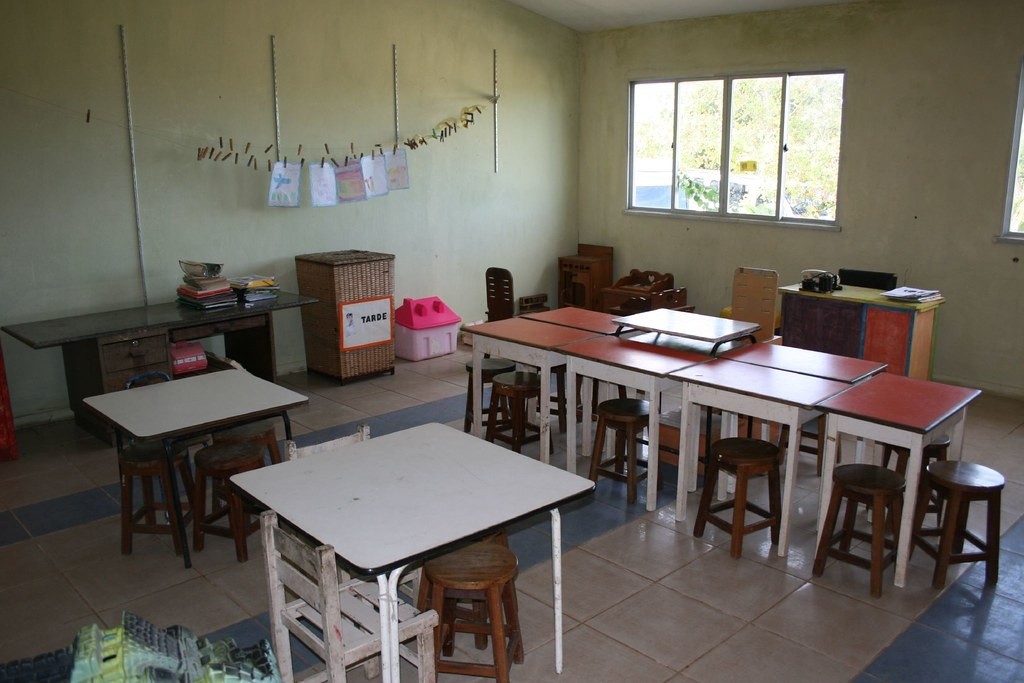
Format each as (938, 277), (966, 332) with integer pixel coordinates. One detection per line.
(879, 286), (942, 303)
(175, 274), (280, 309)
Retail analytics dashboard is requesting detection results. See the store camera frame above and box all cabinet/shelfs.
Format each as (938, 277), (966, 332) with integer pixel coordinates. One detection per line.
(0, 288), (320, 448)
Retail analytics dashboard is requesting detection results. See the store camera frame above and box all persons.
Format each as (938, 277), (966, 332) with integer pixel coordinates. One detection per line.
(345, 312), (356, 337)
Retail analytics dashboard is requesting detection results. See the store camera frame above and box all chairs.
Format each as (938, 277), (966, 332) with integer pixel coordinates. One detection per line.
(127, 370), (219, 522)
(256, 510), (439, 683)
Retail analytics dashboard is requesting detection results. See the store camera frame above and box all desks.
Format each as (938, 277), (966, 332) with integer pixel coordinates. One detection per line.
(461, 306), (981, 588)
(230, 422), (596, 675)
(777, 278), (948, 382)
(81, 369), (309, 569)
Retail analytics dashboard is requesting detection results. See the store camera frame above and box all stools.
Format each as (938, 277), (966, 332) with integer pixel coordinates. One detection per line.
(484, 372), (556, 456)
(586, 399), (664, 503)
(463, 358), (515, 433)
(803, 429), (1005, 597)
(418, 542), (524, 683)
(692, 437), (783, 559)
(116, 423), (284, 563)
